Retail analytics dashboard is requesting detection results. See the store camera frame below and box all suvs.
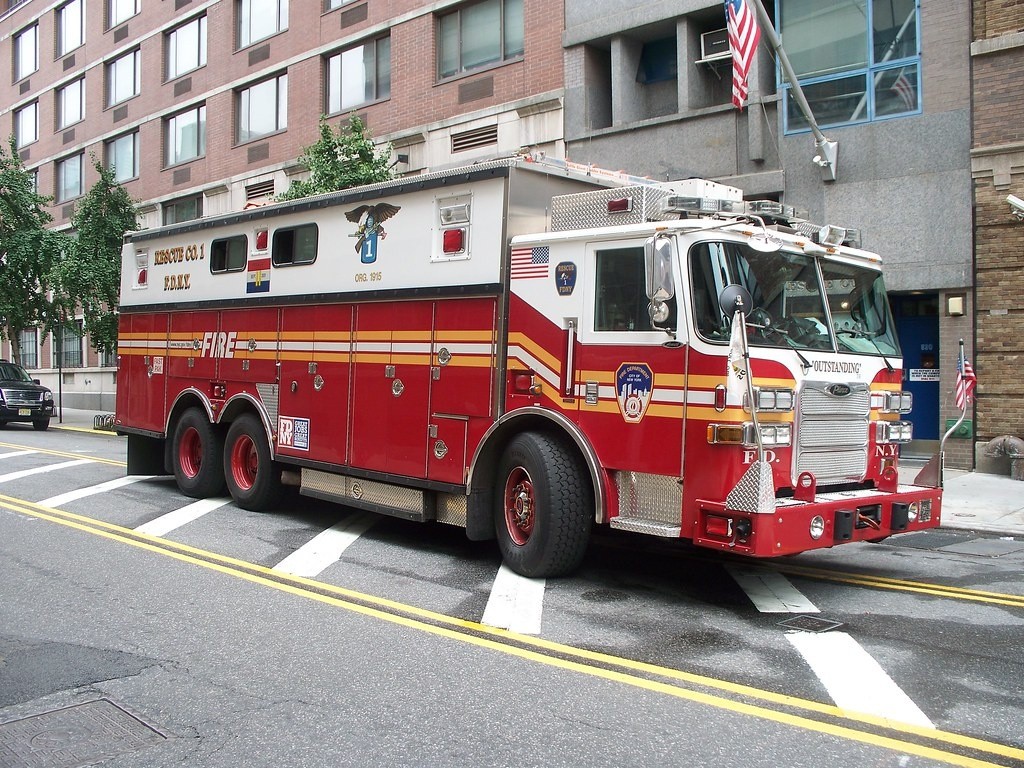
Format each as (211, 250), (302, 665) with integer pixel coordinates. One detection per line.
(1, 358), (55, 434)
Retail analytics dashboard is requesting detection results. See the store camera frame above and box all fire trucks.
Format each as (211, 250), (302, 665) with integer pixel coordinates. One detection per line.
(108, 147), (944, 582)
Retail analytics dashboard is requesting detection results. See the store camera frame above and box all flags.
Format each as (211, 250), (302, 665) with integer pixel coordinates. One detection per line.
(891, 39), (916, 114)
(720, 0), (761, 111)
(954, 345), (979, 411)
